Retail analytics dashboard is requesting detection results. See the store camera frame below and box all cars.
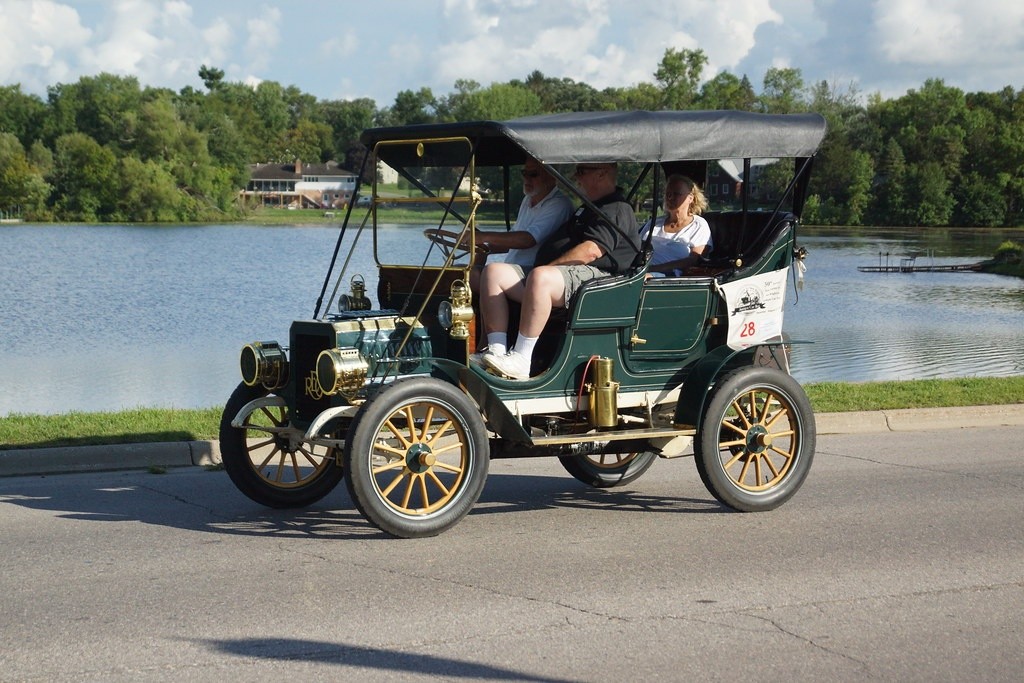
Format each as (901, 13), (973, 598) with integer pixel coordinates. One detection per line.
(216, 106), (832, 543)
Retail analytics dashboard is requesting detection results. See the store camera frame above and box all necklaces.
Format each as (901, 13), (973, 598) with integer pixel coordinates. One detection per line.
(669, 213), (685, 229)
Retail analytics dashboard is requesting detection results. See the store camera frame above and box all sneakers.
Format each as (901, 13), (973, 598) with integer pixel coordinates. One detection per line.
(468, 344), (498, 370)
(482, 346), (530, 379)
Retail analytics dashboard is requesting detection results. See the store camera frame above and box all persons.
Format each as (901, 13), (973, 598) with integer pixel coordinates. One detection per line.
(467, 160), (643, 382)
(639, 173), (715, 282)
(455, 155), (575, 351)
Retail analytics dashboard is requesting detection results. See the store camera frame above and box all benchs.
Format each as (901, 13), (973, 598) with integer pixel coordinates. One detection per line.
(628, 209), (798, 360)
(472, 240), (655, 333)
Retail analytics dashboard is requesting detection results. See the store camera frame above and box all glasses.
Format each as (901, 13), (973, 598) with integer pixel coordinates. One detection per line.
(663, 192), (690, 198)
(521, 170), (546, 178)
(576, 166), (602, 175)
